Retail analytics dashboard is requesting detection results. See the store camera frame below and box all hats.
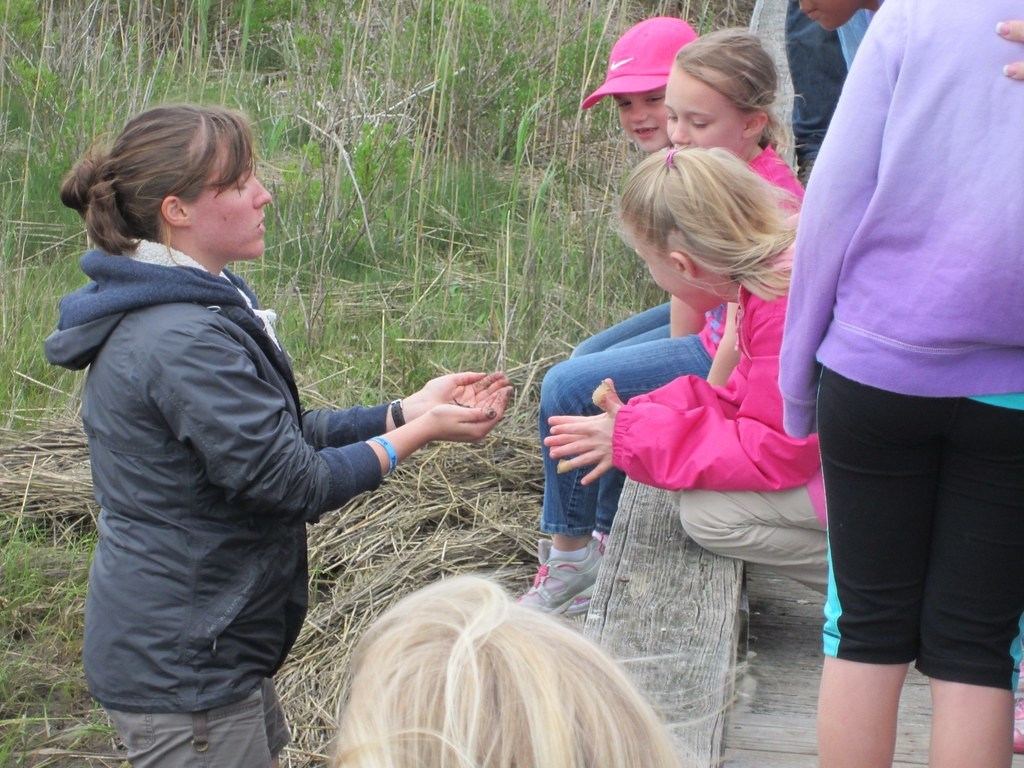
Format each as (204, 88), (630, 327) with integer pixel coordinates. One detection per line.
(583, 16), (697, 106)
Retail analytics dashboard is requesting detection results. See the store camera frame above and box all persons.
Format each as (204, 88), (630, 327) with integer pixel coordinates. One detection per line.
(43, 108), (512, 768)
(517, 27), (807, 617)
(782, 0), (1024, 768)
(543, 146), (828, 598)
(332, 575), (678, 768)
(572, 17), (703, 360)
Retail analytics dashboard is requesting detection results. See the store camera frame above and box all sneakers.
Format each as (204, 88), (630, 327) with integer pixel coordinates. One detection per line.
(514, 534), (611, 619)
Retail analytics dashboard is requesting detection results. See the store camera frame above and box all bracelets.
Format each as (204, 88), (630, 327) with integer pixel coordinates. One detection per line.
(369, 437), (397, 478)
(391, 398), (405, 429)
